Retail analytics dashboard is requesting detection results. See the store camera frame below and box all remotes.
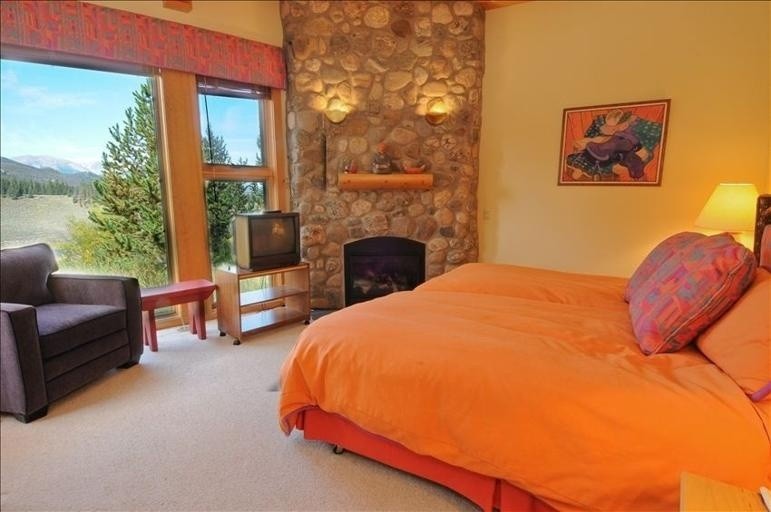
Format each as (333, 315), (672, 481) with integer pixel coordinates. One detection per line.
(264, 210), (281, 214)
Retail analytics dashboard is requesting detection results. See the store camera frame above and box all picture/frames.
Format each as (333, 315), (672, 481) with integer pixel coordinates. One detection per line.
(553, 97), (672, 188)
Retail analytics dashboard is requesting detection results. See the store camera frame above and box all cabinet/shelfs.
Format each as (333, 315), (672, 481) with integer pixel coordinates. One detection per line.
(214, 260), (315, 345)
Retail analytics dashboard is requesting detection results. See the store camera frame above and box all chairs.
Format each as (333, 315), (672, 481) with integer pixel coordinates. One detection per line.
(1, 238), (149, 426)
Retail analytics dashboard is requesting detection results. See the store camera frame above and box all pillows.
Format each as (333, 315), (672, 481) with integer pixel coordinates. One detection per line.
(623, 228), (705, 302)
(626, 230), (759, 358)
(692, 270), (771, 401)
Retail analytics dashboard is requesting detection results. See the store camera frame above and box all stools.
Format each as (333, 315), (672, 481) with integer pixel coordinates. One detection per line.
(138, 276), (220, 354)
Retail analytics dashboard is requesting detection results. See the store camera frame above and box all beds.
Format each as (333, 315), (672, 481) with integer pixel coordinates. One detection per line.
(269, 186), (771, 510)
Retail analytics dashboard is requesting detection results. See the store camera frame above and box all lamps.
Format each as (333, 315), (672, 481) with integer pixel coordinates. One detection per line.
(695, 182), (765, 242)
(325, 93), (351, 126)
(424, 93), (450, 126)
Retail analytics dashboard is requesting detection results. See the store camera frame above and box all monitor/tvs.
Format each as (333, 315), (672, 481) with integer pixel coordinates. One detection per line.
(230, 211), (300, 272)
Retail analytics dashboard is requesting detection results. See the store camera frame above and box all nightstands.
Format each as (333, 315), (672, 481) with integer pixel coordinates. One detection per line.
(672, 465), (770, 511)
